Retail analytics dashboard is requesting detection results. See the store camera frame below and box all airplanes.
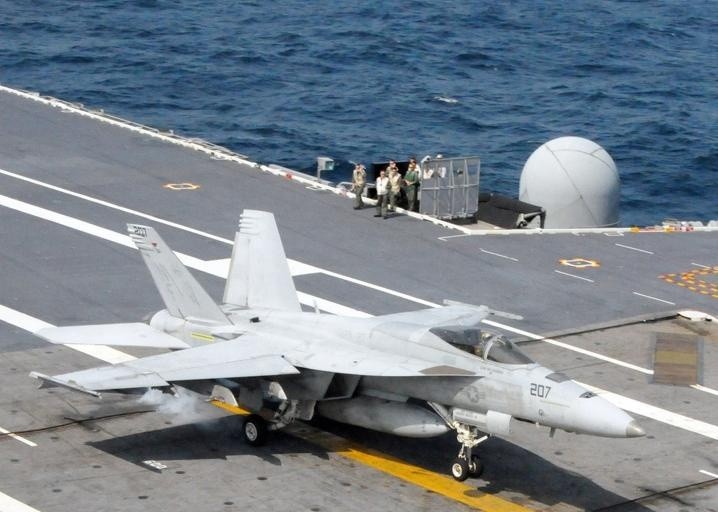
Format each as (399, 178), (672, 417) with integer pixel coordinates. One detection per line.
(28, 208), (647, 483)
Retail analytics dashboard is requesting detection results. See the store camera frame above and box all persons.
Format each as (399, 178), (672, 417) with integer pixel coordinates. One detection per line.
(403, 153), (447, 211)
(350, 163), (367, 209)
(385, 160), (398, 204)
(386, 167), (402, 212)
(374, 169), (393, 219)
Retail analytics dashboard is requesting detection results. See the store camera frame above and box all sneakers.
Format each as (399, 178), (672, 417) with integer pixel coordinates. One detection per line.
(374, 209), (397, 219)
